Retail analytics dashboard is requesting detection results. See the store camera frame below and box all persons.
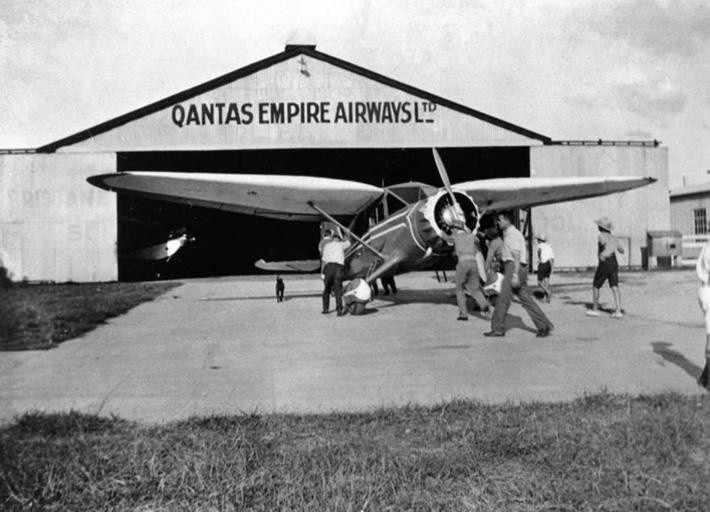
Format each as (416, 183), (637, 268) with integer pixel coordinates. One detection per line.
(535, 233), (554, 303)
(483, 209), (554, 338)
(479, 227), (505, 274)
(441, 220), (490, 320)
(585, 216), (624, 318)
(318, 229), (398, 317)
(696, 219), (710, 391)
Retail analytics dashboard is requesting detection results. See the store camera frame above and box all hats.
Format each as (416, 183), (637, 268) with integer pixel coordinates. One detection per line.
(485, 227), (499, 240)
(452, 219), (464, 229)
(593, 215), (615, 232)
(535, 232), (547, 242)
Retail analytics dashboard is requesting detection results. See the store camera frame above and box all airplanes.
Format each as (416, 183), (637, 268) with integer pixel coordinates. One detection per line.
(86, 148), (658, 315)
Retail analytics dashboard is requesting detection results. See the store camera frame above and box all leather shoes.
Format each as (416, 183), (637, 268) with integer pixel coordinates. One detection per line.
(321, 309), (343, 316)
(457, 305), (490, 321)
(536, 322), (555, 337)
(483, 330), (505, 337)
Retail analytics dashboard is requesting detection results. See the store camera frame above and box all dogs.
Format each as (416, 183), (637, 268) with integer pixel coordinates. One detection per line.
(276, 275), (284, 304)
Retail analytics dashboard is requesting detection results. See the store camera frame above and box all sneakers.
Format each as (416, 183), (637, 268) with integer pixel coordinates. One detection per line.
(585, 310), (623, 318)
